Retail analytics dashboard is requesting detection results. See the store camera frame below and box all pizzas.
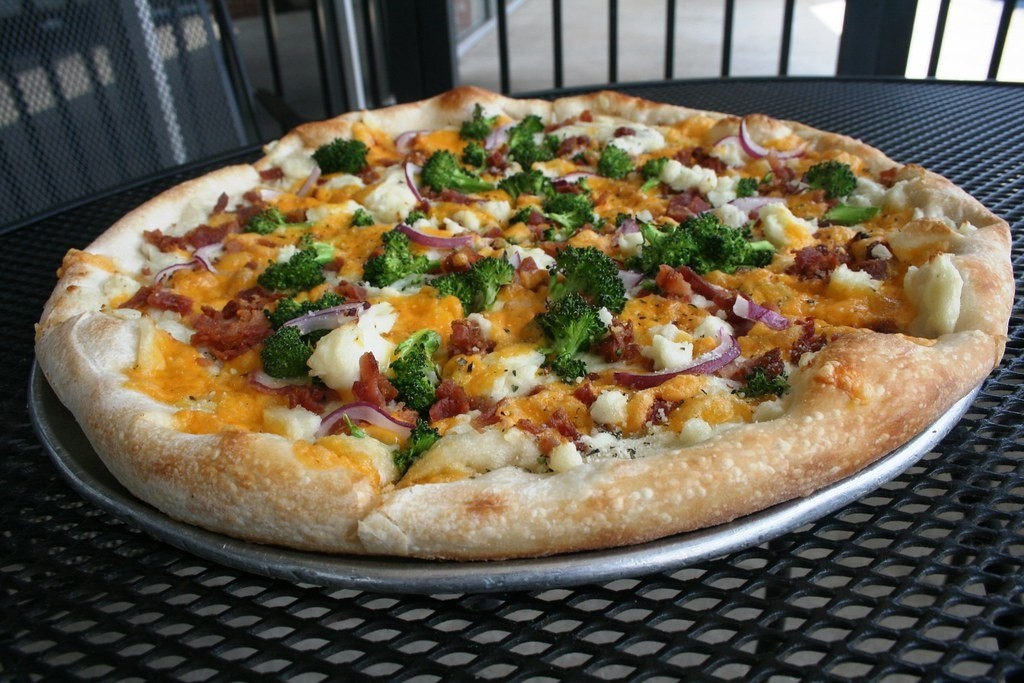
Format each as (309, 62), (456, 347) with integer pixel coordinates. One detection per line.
(34, 85), (1014, 561)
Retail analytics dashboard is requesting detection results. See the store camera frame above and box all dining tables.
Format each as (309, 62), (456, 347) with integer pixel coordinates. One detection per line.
(0, 77), (1024, 683)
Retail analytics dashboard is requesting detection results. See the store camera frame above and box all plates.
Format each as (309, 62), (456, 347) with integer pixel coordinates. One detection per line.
(31, 360), (983, 591)
